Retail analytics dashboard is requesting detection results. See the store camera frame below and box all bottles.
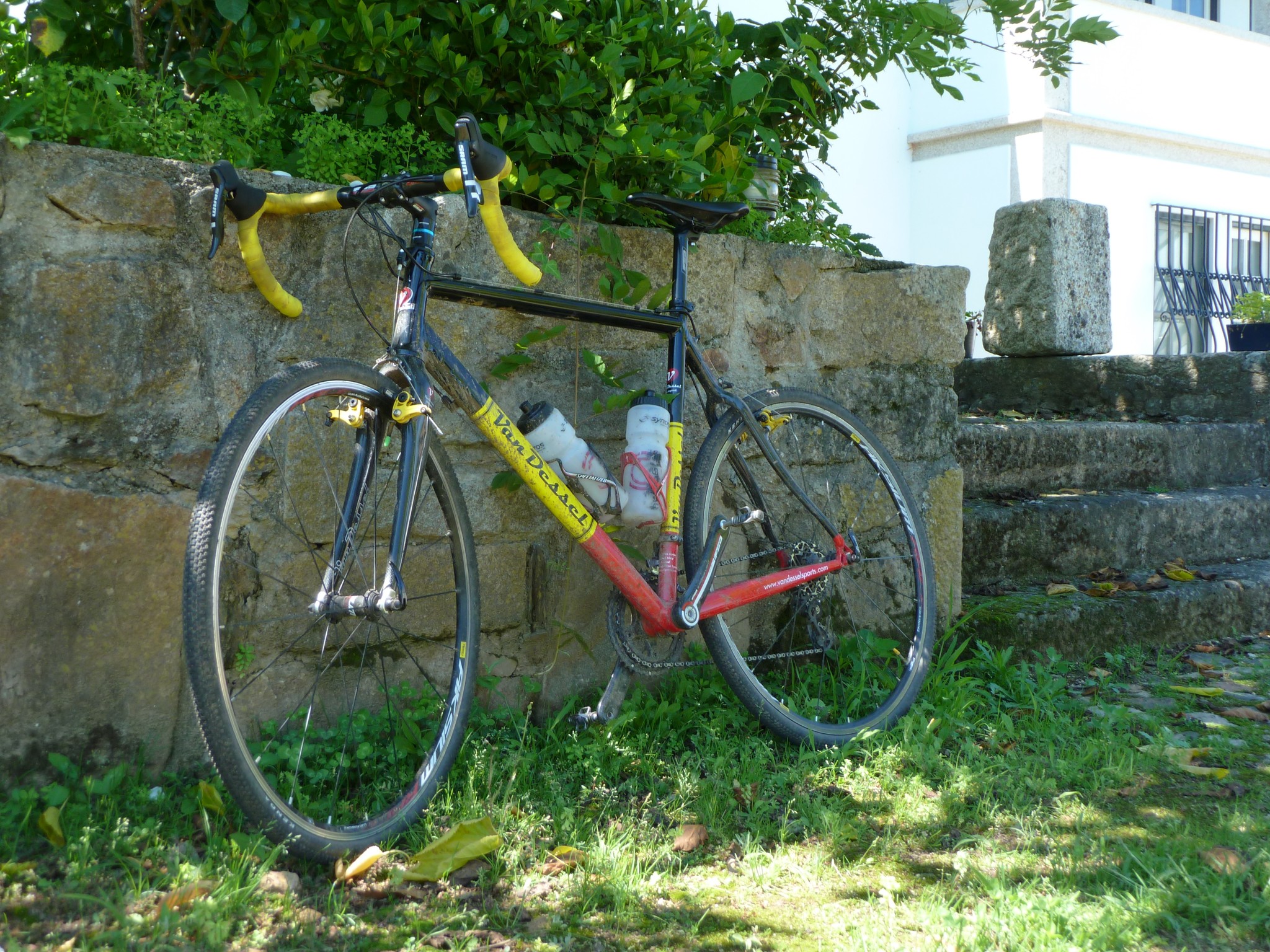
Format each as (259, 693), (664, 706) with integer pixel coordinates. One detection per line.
(619, 392), (670, 528)
(516, 400), (629, 523)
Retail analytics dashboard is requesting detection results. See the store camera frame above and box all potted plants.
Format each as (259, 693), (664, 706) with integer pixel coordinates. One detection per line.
(964, 309), (984, 359)
(1224, 291), (1270, 353)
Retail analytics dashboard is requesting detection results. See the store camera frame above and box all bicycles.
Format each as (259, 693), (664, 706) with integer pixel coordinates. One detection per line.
(180, 109), (943, 856)
